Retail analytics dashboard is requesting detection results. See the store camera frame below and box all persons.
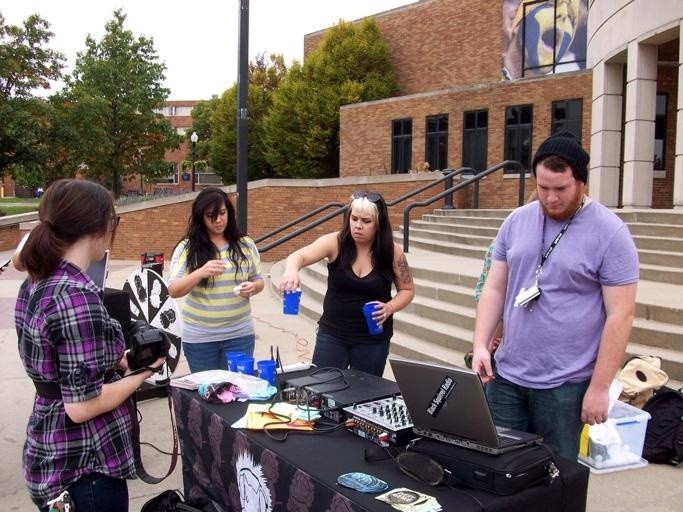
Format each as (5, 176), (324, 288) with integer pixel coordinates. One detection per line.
(477, 189), (542, 350)
(504, 0), (581, 80)
(11, 222), (35, 273)
(0, 263), (9, 276)
(168, 186), (265, 372)
(471, 130), (641, 460)
(279, 191), (416, 379)
(14, 178), (166, 512)
(416, 162), (433, 173)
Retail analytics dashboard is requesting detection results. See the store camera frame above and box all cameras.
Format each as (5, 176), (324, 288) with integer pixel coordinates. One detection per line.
(126, 320), (165, 371)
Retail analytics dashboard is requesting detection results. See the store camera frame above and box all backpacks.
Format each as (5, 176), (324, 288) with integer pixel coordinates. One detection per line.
(617, 355), (669, 409)
(642, 386), (682, 465)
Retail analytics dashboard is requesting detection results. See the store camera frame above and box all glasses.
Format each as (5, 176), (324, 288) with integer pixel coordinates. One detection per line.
(353, 191), (381, 203)
(109, 215), (119, 232)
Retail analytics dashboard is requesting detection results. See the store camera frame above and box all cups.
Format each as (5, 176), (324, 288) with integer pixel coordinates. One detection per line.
(282, 288), (302, 315)
(226, 352), (277, 386)
(363, 303), (384, 336)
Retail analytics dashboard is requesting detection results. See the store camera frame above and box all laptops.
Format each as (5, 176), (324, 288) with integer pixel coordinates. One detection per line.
(389, 358), (544, 455)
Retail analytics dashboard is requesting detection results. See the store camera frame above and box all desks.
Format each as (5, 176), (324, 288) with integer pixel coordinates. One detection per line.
(167, 364), (590, 512)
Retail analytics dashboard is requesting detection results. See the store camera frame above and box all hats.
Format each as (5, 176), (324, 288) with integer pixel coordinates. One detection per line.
(532, 130), (590, 185)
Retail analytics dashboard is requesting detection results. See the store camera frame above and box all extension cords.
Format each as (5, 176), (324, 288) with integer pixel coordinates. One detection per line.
(275, 362), (310, 375)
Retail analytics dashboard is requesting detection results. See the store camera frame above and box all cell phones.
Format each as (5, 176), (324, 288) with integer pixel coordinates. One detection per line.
(516, 287), (540, 306)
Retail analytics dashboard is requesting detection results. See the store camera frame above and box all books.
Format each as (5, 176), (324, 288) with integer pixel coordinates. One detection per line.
(171, 368), (230, 390)
(245, 398), (313, 432)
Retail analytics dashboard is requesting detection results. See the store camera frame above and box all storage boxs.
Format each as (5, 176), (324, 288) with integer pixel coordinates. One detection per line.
(577, 399), (651, 474)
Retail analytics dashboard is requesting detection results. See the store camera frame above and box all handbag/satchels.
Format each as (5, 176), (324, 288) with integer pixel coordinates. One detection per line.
(364, 436), (559, 495)
